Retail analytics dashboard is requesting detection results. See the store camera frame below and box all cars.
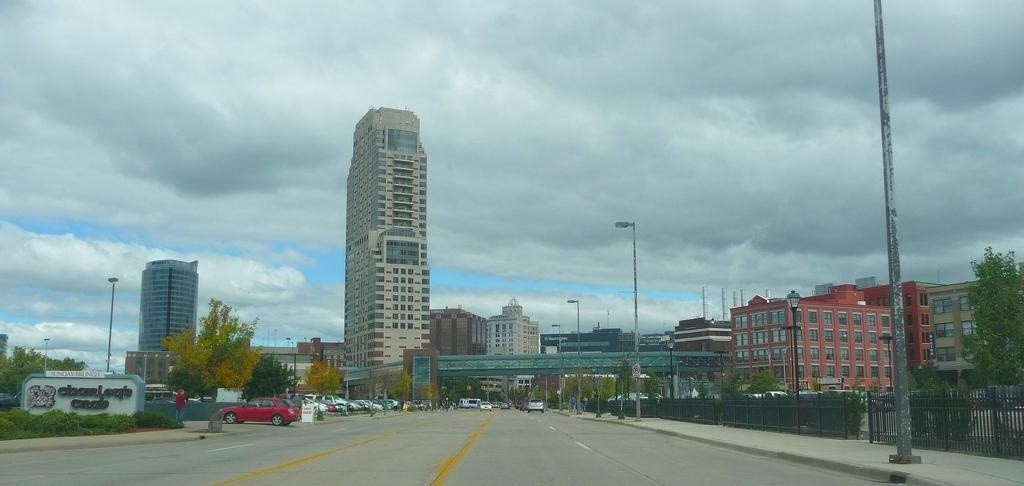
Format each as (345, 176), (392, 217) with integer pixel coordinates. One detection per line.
(458, 397), (532, 412)
(0, 392), (13, 407)
(11, 390), (21, 407)
(607, 391), (664, 402)
(299, 394), (427, 412)
(145, 390), (215, 403)
(216, 396), (302, 427)
(746, 390), (1024, 411)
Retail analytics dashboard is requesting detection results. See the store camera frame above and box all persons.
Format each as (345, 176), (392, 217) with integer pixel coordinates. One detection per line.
(417, 401), (454, 416)
(176, 389), (186, 424)
(293, 393), (301, 407)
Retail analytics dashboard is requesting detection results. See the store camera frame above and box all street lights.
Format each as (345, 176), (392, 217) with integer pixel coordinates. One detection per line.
(105, 276), (120, 371)
(878, 334), (894, 392)
(592, 365), (596, 393)
(551, 323), (562, 352)
(782, 324), (802, 394)
(785, 288), (804, 435)
(143, 353), (147, 383)
(714, 350), (729, 383)
(668, 336), (675, 399)
(615, 219), (645, 421)
(567, 298), (586, 415)
(42, 337), (51, 371)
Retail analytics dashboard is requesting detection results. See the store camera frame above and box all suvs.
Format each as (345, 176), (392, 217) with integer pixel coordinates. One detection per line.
(528, 398), (545, 413)
